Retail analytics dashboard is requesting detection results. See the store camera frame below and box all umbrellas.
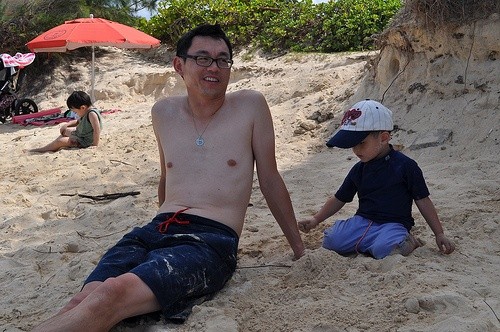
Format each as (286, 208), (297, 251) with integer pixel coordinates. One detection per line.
(25, 14), (161, 106)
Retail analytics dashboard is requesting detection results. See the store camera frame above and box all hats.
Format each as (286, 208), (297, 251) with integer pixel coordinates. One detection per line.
(326, 98), (394, 148)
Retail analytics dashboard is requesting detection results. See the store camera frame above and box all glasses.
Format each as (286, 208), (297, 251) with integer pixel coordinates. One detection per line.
(179, 55), (233, 69)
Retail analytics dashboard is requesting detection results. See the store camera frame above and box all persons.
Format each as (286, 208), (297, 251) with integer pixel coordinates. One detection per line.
(22, 91), (103, 152)
(30, 23), (306, 332)
(298, 98), (455, 259)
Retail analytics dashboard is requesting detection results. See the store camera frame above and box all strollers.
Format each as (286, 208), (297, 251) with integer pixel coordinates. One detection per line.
(0, 53), (39, 123)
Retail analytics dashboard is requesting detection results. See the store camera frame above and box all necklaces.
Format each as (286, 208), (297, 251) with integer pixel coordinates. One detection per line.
(187, 97), (222, 146)
